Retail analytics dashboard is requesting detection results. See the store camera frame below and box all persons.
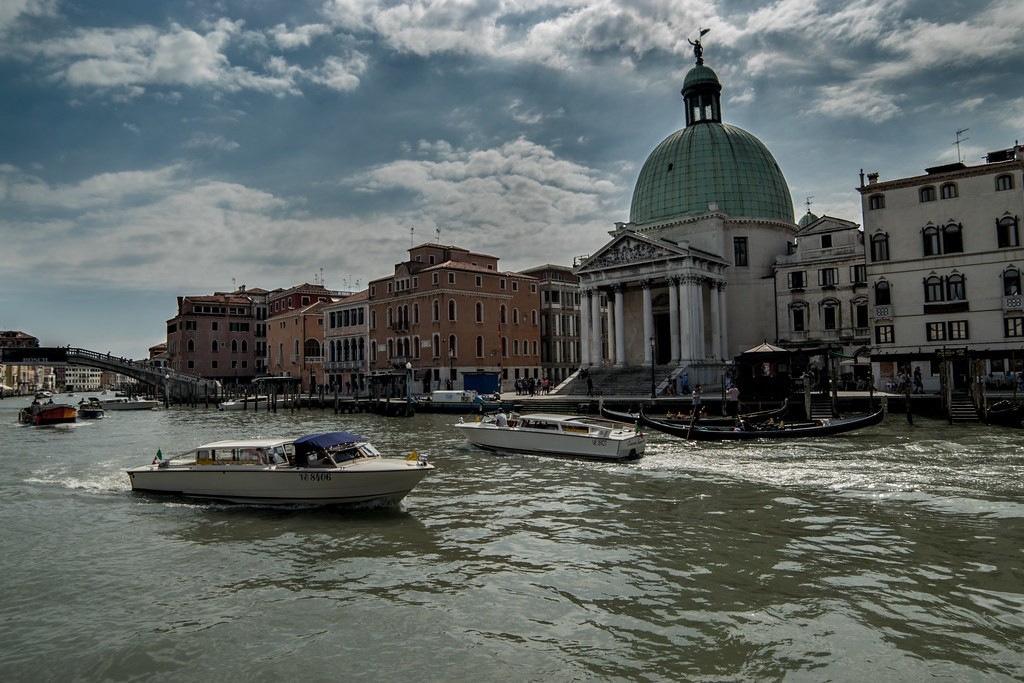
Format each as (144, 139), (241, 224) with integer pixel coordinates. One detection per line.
(67, 344), (70, 354)
(691, 385), (703, 420)
(325, 384), (329, 395)
(107, 350), (202, 382)
(726, 383), (740, 418)
(679, 373), (692, 395)
(867, 371), (877, 393)
(78, 398), (86, 405)
(446, 379), (451, 390)
(495, 408), (507, 426)
(895, 370), (909, 393)
(665, 374), (674, 396)
(984, 373), (991, 390)
(913, 366), (924, 394)
(587, 375), (594, 398)
(514, 377), (554, 397)
(493, 390), (501, 400)
(817, 371), (823, 394)
(1004, 370), (1024, 392)
(47, 399), (53, 405)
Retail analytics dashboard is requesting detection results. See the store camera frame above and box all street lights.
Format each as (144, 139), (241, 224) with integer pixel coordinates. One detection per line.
(649, 334), (658, 398)
(448, 348), (453, 390)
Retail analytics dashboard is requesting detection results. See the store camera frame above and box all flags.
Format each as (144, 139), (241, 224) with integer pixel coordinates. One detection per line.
(152, 447), (162, 464)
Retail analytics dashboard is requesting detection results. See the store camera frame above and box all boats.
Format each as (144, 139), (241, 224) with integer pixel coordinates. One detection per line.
(34, 388), (53, 399)
(218, 394), (297, 410)
(99, 397), (160, 410)
(986, 399), (1024, 425)
(125, 430), (436, 509)
(600, 398), (792, 425)
(18, 402), (77, 425)
(68, 391), (75, 396)
(454, 410), (650, 459)
(637, 394), (889, 439)
(77, 397), (105, 418)
